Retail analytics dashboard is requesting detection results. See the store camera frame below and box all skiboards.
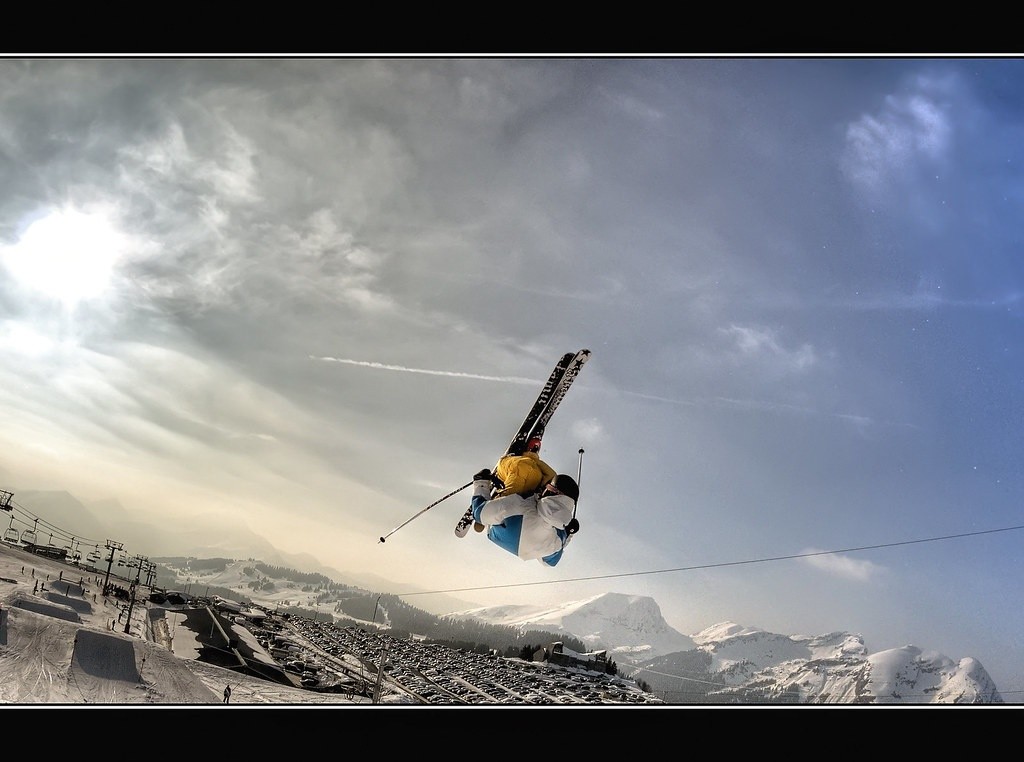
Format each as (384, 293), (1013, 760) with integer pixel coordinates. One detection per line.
(454, 347), (595, 541)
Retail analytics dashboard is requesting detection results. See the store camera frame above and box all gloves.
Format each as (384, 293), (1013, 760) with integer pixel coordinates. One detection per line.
(474, 469), (491, 481)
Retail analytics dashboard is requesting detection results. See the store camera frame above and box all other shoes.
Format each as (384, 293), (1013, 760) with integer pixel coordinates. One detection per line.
(528, 439), (541, 453)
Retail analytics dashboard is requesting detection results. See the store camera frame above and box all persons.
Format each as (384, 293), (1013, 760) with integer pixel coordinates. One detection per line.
(223, 685), (231, 704)
(73, 553), (80, 560)
(471, 433), (579, 567)
(22, 566), (125, 630)
